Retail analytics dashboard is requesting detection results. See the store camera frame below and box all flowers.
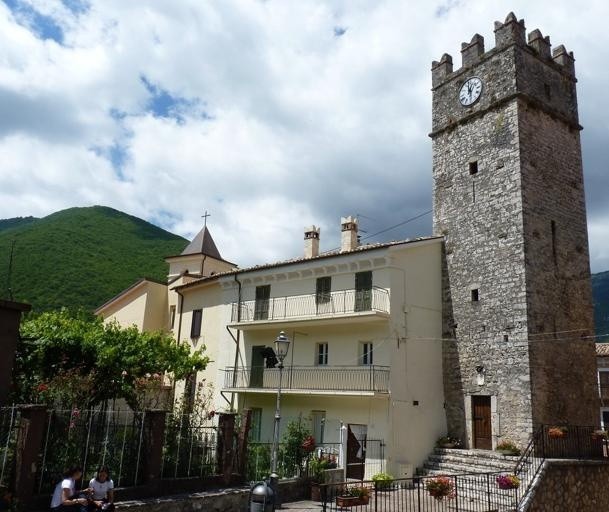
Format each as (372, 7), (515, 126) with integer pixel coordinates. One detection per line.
(548, 425), (569, 438)
(426, 476), (458, 501)
(495, 473), (520, 487)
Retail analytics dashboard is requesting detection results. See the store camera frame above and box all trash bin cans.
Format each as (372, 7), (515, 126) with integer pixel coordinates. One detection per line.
(248, 482), (275, 512)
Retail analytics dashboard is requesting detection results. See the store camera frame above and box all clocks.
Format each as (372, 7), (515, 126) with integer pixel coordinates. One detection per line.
(458, 76), (483, 107)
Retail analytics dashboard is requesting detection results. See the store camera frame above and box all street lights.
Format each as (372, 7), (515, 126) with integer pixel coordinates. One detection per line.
(268, 330), (292, 509)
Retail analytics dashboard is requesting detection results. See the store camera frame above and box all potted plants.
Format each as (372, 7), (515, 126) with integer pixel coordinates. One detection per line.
(372, 472), (394, 488)
(336, 486), (371, 508)
(494, 440), (522, 454)
(309, 457), (330, 501)
(435, 435), (463, 448)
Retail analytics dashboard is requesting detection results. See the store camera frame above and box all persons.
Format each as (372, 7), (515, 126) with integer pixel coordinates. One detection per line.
(88, 466), (116, 512)
(50, 468), (95, 512)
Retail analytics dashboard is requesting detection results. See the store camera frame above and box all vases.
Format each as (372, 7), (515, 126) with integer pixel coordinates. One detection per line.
(548, 434), (566, 439)
(429, 487), (449, 496)
(499, 483), (518, 489)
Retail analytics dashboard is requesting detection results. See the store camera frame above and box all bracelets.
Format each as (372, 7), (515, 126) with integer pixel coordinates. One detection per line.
(73, 499), (78, 504)
